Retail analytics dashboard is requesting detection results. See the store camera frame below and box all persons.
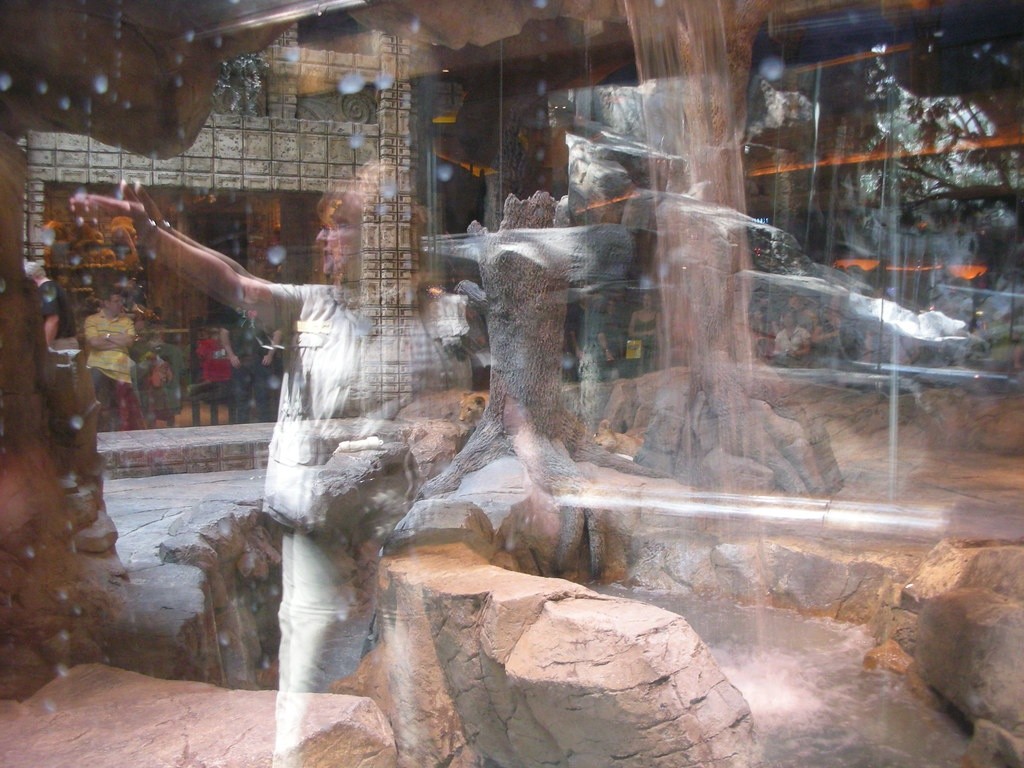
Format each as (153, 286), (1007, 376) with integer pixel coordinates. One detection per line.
(22, 244), (864, 429)
(70, 169), (479, 768)
